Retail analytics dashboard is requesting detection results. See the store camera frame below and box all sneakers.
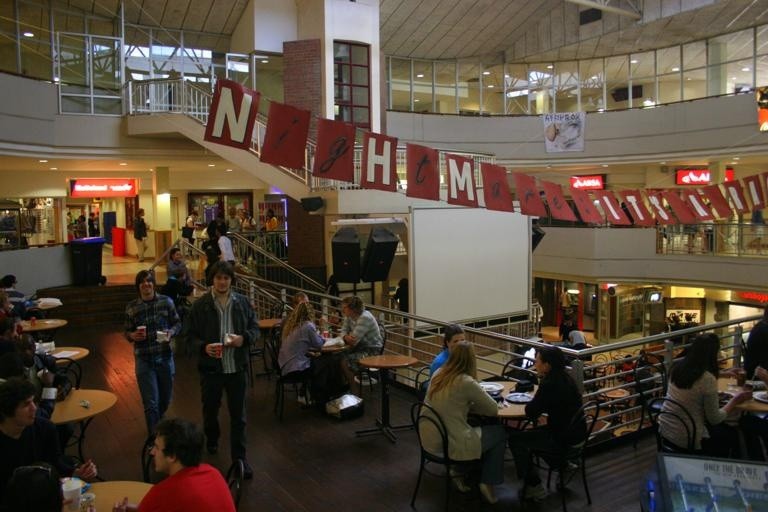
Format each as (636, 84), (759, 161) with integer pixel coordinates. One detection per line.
(517, 485), (547, 501)
(479, 483), (498, 504)
(449, 470), (471, 492)
(555, 461), (577, 489)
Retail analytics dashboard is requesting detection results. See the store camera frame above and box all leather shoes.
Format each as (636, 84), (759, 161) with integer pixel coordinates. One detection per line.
(244, 463), (253, 480)
(207, 443), (218, 454)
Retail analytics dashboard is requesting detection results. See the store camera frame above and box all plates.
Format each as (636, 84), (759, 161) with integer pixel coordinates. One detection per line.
(506, 392), (532, 404)
(477, 382), (504, 391)
(753, 391), (767, 405)
(717, 390), (737, 402)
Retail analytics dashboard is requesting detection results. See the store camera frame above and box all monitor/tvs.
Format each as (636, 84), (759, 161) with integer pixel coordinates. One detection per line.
(650, 293), (661, 302)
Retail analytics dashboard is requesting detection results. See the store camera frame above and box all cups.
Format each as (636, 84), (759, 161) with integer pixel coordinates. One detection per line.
(136, 326), (147, 339)
(213, 342), (223, 360)
(62, 479), (82, 511)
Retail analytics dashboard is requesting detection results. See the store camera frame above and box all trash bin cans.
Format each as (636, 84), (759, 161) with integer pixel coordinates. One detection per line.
(62, 236), (107, 288)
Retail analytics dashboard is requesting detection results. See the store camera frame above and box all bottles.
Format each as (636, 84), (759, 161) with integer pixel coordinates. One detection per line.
(323, 330), (328, 339)
(490, 391), (504, 403)
(37, 340), (44, 355)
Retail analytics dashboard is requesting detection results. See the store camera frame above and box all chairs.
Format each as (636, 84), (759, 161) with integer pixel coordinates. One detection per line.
(408, 363), (600, 512)
(251, 298), (388, 418)
(646, 392), (699, 456)
(591, 347), (654, 437)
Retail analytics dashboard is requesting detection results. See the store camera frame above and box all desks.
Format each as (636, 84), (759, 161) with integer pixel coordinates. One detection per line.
(354, 354), (420, 445)
(63, 478), (154, 512)
(19, 299), (119, 430)
(711, 376), (767, 427)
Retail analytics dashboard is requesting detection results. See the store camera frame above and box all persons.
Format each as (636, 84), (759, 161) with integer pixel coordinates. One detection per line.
(0, 201), (407, 511)
(429, 323), (466, 386)
(508, 347), (587, 501)
(613, 197), (768, 256)
(530, 285), (702, 392)
(743, 305), (768, 376)
(658, 333), (752, 459)
(738, 364), (768, 461)
(418, 341), (499, 505)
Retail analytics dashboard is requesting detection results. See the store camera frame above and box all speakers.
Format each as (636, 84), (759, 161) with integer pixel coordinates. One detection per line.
(331, 227), (360, 283)
(300, 196), (324, 212)
(362, 226), (399, 283)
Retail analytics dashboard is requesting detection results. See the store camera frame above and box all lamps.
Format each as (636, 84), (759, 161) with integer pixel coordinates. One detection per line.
(156, 186), (174, 196)
